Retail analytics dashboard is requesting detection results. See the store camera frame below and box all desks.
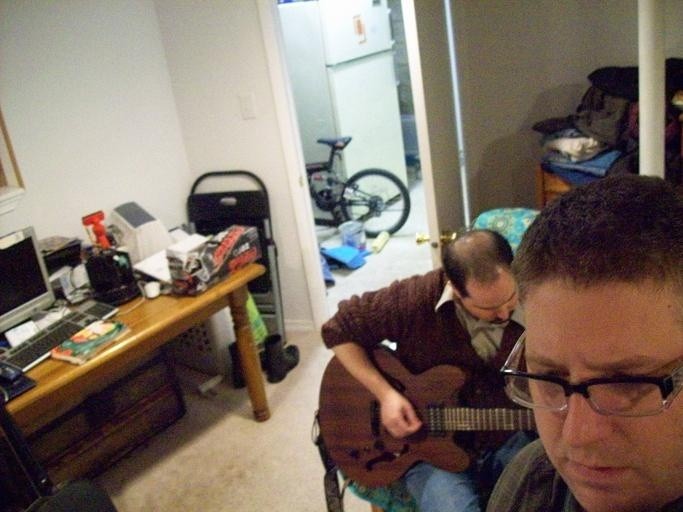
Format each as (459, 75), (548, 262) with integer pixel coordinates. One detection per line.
(3, 262), (272, 440)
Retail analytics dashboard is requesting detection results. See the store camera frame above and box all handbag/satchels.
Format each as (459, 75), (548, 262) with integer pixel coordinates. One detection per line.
(572, 85), (632, 147)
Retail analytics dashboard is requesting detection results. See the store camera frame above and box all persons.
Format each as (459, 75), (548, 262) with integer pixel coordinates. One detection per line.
(482, 172), (683, 512)
(319, 226), (542, 512)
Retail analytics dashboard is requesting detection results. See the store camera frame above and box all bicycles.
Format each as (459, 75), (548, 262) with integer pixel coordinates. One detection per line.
(305, 136), (410, 239)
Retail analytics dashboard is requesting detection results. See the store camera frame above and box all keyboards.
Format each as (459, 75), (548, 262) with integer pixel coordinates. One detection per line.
(1, 301), (119, 374)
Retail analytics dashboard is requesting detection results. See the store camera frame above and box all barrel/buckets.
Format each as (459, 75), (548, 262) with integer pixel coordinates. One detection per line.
(339, 220), (367, 254)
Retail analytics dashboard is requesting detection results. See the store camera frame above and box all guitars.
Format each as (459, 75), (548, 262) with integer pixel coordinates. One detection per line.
(318, 345), (538, 489)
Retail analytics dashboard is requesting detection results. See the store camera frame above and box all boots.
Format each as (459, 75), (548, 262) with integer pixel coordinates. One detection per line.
(227, 341), (264, 387)
(264, 333), (299, 384)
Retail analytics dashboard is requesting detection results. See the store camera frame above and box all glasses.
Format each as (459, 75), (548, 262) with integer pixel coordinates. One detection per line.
(498, 332), (683, 418)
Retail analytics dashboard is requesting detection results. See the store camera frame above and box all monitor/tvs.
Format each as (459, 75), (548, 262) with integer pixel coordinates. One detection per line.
(0, 225), (57, 336)
